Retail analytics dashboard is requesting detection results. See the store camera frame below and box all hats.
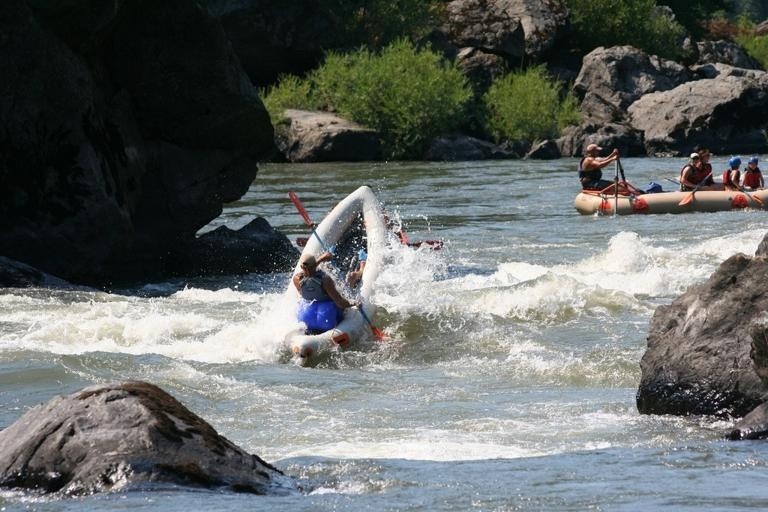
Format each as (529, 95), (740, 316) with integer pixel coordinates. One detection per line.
(299, 253), (317, 270)
(690, 149), (711, 159)
(586, 144), (603, 153)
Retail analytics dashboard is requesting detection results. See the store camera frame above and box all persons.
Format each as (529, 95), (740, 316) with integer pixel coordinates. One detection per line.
(679, 153), (717, 190)
(578, 144), (641, 195)
(699, 148), (714, 185)
(291, 247), (366, 311)
(722, 157), (744, 190)
(740, 158), (765, 191)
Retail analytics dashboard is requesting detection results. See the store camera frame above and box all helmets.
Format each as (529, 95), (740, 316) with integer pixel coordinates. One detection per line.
(748, 156), (758, 165)
(729, 156), (742, 166)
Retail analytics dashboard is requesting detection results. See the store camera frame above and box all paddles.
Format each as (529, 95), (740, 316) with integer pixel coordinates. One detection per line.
(678, 171), (715, 207)
(288, 191), (385, 341)
(741, 190), (765, 206)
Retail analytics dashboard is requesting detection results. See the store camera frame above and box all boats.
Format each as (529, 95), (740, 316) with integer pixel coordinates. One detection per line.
(574, 179), (768, 214)
(281, 185), (386, 370)
